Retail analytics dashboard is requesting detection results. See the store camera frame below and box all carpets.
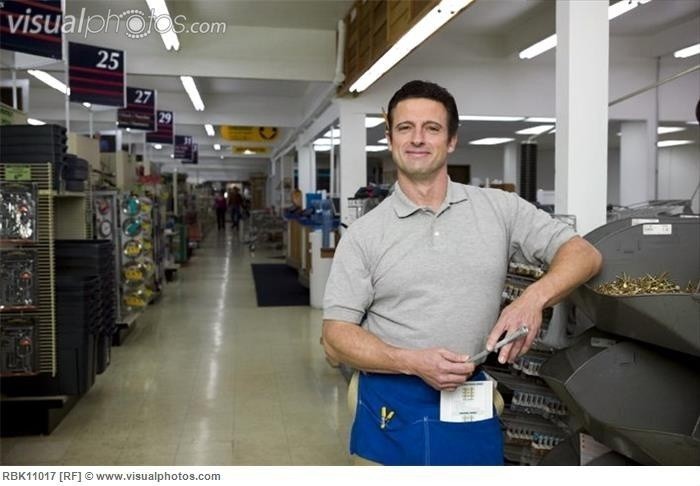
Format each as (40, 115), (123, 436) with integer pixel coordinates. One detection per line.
(250, 263), (311, 307)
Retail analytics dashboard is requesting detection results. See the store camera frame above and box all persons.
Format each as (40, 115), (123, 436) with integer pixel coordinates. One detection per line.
(320, 79), (603, 466)
(212, 187), (244, 230)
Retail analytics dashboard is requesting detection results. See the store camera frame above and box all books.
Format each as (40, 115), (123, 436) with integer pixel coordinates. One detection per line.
(440, 380), (494, 423)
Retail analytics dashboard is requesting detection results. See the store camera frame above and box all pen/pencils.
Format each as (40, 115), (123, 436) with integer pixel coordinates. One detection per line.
(380, 407), (395, 430)
(464, 326), (529, 364)
